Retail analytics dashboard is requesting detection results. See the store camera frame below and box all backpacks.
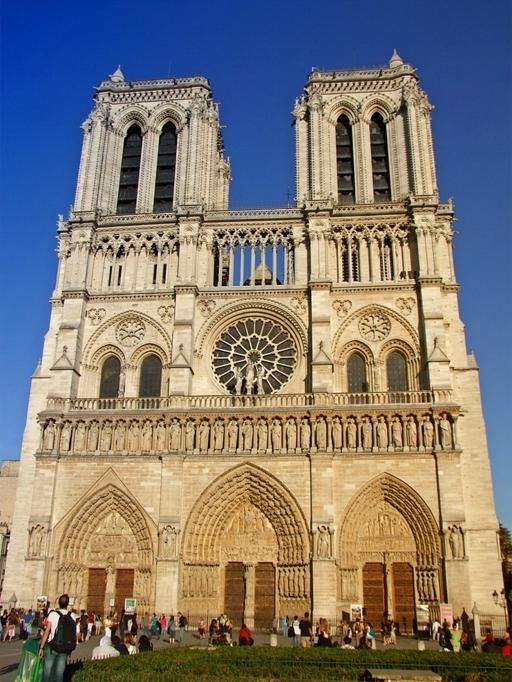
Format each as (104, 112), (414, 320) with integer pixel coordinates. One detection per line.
(366, 627), (374, 635)
(288, 622), (295, 637)
(131, 622), (137, 634)
(46, 611), (77, 654)
(439, 631), (449, 647)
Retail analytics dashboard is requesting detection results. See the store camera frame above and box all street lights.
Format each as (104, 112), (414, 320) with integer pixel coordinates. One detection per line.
(491, 587), (511, 634)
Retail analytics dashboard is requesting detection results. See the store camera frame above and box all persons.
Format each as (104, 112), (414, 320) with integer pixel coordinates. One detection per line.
(207, 612), (235, 647)
(238, 623), (251, 646)
(272, 610), (511, 658)
(0, 604), (187, 656)
(198, 616), (207, 640)
(36, 594), (77, 681)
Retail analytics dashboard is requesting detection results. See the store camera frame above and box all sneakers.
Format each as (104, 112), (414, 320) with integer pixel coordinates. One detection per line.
(2, 640), (12, 643)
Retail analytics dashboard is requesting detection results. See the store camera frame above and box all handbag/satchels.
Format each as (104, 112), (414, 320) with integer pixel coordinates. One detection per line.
(168, 626), (173, 635)
(9, 629), (15, 636)
(182, 617), (187, 626)
